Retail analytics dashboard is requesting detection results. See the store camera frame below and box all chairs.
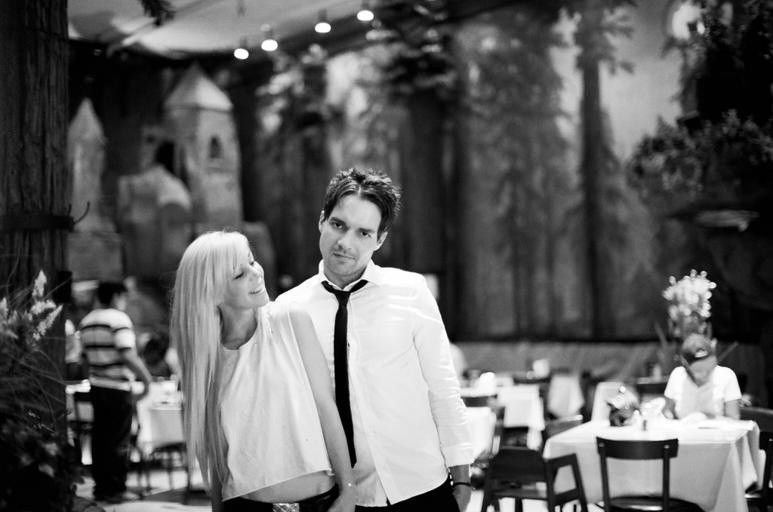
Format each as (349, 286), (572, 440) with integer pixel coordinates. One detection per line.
(461, 367), (773, 511)
(68, 389), (194, 505)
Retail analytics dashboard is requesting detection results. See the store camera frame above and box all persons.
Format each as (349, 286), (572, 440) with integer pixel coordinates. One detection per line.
(655, 332), (743, 423)
(159, 226), (357, 512)
(75, 280), (154, 505)
(270, 160), (476, 512)
(64, 315), (84, 383)
(131, 329), (174, 384)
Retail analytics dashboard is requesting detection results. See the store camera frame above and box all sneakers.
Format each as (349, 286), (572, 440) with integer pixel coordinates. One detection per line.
(94, 486), (141, 503)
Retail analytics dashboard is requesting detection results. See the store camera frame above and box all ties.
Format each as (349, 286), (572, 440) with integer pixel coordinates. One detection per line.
(322, 279), (370, 468)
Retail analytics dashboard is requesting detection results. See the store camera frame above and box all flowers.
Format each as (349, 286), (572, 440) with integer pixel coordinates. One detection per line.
(662, 268), (717, 362)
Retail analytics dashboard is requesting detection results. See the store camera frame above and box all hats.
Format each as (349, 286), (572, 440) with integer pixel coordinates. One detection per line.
(680, 333), (718, 366)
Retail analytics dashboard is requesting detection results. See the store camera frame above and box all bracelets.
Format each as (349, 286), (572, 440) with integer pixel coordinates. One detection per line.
(338, 478), (357, 490)
(452, 480), (473, 489)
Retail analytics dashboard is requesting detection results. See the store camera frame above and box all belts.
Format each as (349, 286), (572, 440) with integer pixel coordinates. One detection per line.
(220, 482), (340, 512)
